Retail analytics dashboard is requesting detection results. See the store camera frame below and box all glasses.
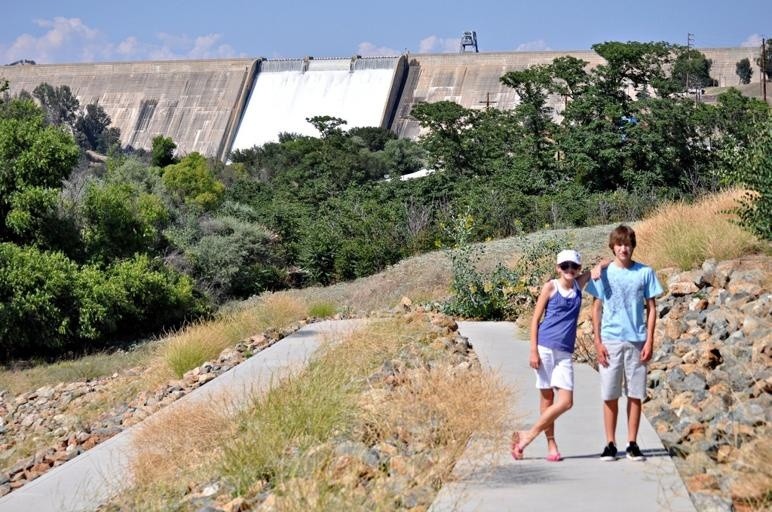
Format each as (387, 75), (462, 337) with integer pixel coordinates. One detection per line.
(560, 262), (579, 270)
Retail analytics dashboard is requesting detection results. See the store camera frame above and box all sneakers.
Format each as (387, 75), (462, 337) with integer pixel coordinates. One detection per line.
(547, 453), (560, 461)
(600, 441), (617, 461)
(512, 432), (523, 459)
(627, 441), (645, 460)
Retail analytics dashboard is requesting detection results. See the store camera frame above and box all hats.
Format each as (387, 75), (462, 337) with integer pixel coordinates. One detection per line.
(557, 250), (580, 265)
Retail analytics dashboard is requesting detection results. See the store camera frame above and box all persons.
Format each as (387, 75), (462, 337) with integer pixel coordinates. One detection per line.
(508, 247), (608, 464)
(584, 223), (664, 464)
